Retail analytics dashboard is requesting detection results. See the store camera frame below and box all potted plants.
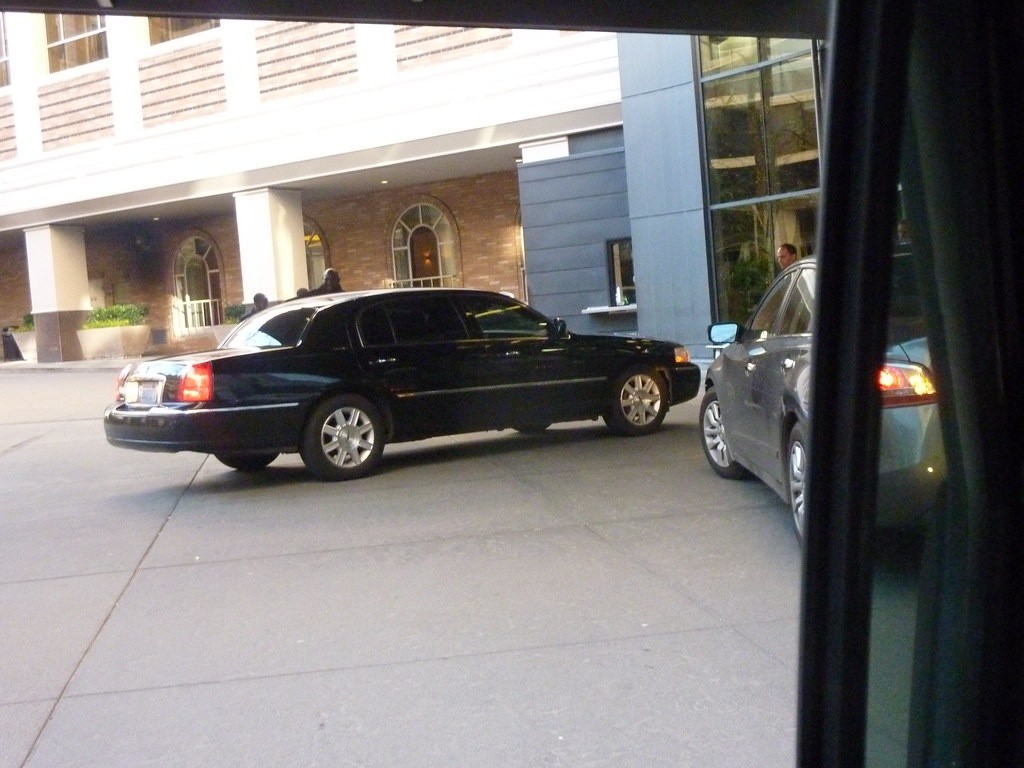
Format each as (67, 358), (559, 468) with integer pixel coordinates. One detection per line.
(212, 303), (245, 344)
(11, 313), (37, 361)
(77, 304), (151, 359)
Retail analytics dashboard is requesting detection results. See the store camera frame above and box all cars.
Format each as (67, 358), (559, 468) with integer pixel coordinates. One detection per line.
(101, 286), (702, 485)
(695, 251), (949, 555)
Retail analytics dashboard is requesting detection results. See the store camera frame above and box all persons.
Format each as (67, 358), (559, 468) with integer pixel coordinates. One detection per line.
(897, 219), (911, 241)
(240, 292), (270, 323)
(297, 288), (308, 296)
(776, 244), (797, 270)
(319, 268), (344, 295)
